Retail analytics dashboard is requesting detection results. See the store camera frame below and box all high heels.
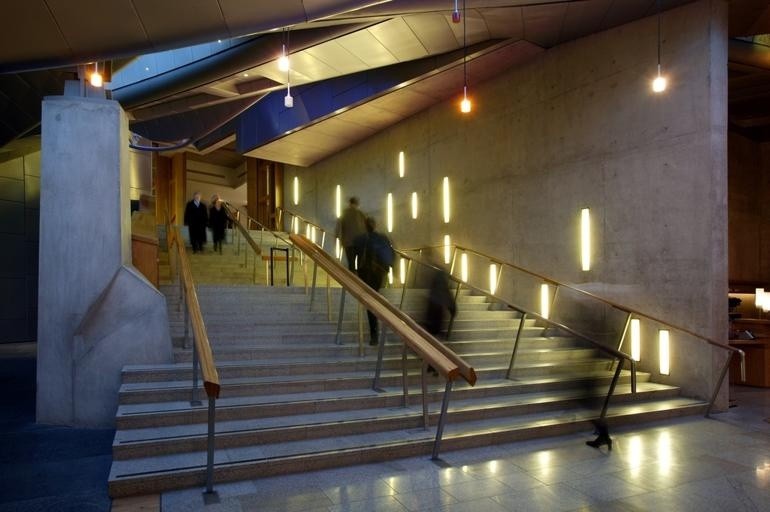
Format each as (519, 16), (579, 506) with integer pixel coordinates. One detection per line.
(585, 437), (612, 452)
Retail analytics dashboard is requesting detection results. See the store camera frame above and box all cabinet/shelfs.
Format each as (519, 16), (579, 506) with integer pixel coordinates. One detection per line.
(726, 283), (769, 387)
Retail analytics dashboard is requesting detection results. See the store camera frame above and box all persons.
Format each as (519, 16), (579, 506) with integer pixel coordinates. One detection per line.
(402, 246), (459, 378)
(184, 191), (209, 256)
(331, 196), (368, 272)
(206, 194), (228, 255)
(585, 417), (613, 451)
(353, 215), (394, 346)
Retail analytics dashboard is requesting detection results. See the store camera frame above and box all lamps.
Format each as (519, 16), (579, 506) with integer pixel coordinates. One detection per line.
(580, 207), (590, 272)
(280, 23), (295, 109)
(399, 257), (406, 284)
(650, 1), (668, 92)
(630, 318), (640, 362)
(386, 192), (393, 233)
(335, 184), (341, 218)
(489, 264), (496, 294)
(443, 234), (450, 264)
(657, 327), (671, 378)
(460, 254), (468, 283)
(290, 215), (326, 250)
(443, 176), (450, 224)
(293, 176), (299, 205)
(541, 283), (549, 321)
(388, 266), (393, 285)
(452, 1), (472, 113)
(335, 238), (358, 271)
(411, 191), (418, 219)
(399, 152), (405, 177)
